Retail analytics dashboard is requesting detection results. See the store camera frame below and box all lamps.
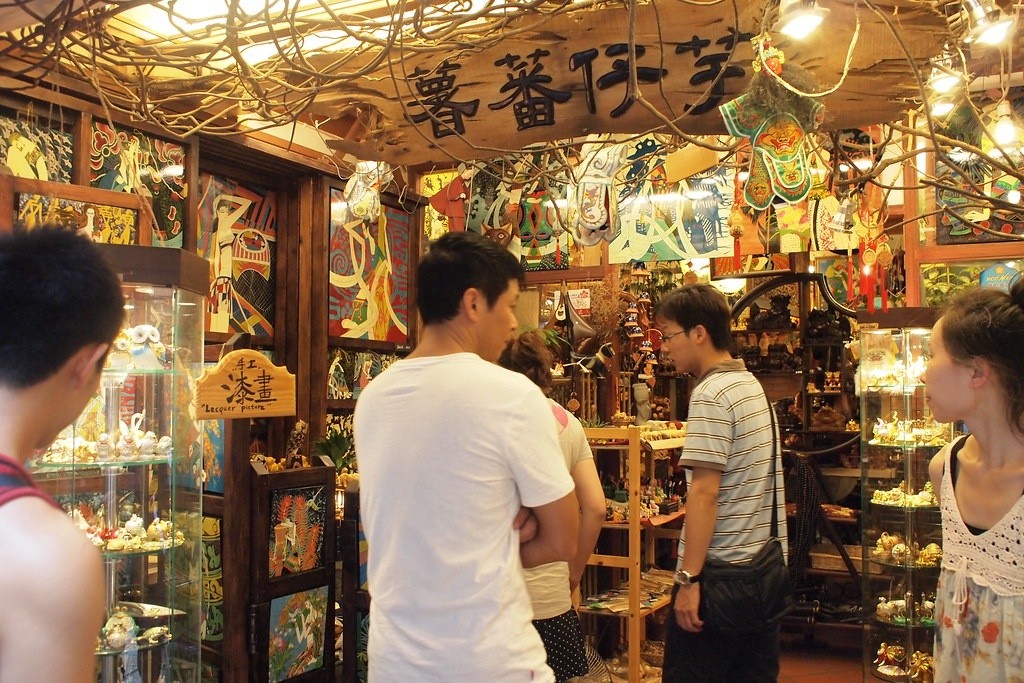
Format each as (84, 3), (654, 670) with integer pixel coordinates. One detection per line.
(960, 0), (1015, 44)
(772, 0), (831, 39)
(926, 61), (959, 116)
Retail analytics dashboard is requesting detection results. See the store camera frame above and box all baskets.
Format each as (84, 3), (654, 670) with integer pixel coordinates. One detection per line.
(617, 640), (665, 667)
(810, 543), (888, 574)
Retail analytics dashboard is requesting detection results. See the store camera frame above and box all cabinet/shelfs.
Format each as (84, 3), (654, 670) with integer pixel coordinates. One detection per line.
(36, 243), (210, 683)
(583, 420), (688, 683)
(856, 306), (963, 683)
(730, 273), (919, 656)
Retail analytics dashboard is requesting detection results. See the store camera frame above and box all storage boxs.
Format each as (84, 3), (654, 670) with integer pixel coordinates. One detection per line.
(808, 544), (887, 575)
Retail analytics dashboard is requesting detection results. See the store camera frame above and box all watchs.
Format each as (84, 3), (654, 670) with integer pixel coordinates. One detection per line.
(675, 569), (701, 586)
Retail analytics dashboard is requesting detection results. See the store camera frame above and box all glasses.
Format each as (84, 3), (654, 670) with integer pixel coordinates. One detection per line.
(661, 329), (687, 343)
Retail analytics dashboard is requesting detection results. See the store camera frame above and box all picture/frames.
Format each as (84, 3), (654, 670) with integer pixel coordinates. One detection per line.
(710, 252), (795, 281)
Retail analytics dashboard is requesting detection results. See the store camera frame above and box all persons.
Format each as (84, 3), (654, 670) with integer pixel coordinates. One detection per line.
(0, 220), (126, 683)
(920, 269), (1024, 683)
(352, 232), (605, 683)
(652, 284), (788, 683)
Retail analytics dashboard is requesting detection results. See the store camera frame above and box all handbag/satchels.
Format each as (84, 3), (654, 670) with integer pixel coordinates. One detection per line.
(697, 536), (797, 634)
